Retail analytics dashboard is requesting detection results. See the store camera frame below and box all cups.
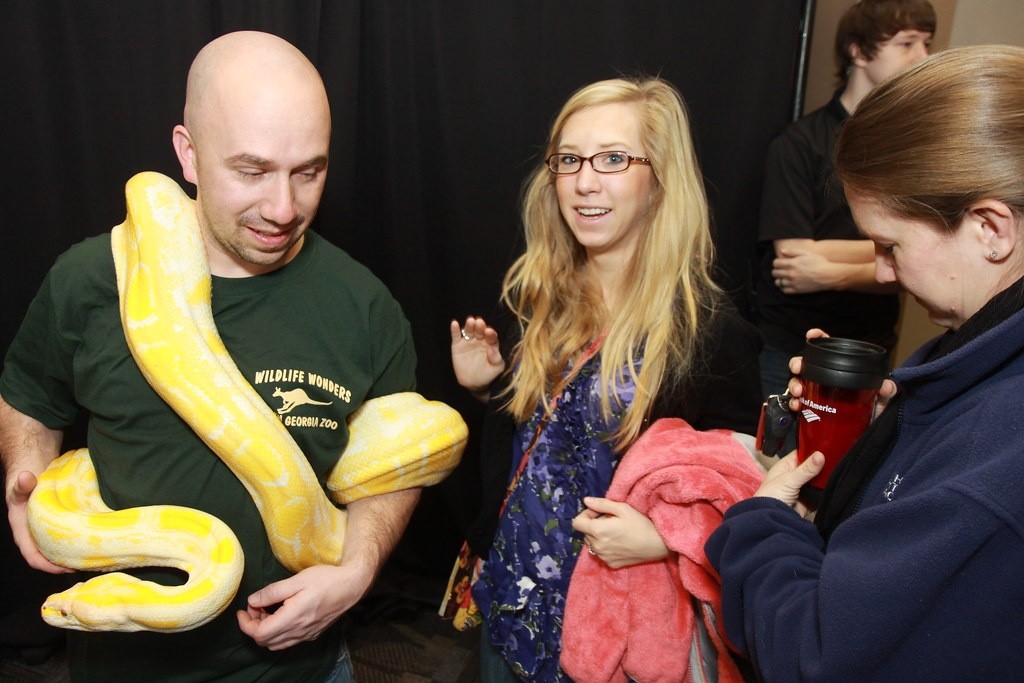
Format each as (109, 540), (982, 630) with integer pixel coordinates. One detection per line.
(797, 337), (887, 506)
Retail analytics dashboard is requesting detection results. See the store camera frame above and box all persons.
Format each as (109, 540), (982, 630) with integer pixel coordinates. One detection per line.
(759, 0), (939, 453)
(704, 44), (1024, 683)
(0, 27), (421, 683)
(451, 76), (779, 683)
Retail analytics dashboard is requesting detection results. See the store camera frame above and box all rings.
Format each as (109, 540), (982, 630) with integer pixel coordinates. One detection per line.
(588, 548), (595, 555)
(461, 329), (470, 340)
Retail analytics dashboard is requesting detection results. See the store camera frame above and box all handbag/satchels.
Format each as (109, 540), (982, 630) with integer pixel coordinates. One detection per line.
(434, 527), (494, 632)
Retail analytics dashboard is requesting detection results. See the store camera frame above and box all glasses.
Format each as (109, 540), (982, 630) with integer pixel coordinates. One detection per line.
(545, 151), (651, 175)
